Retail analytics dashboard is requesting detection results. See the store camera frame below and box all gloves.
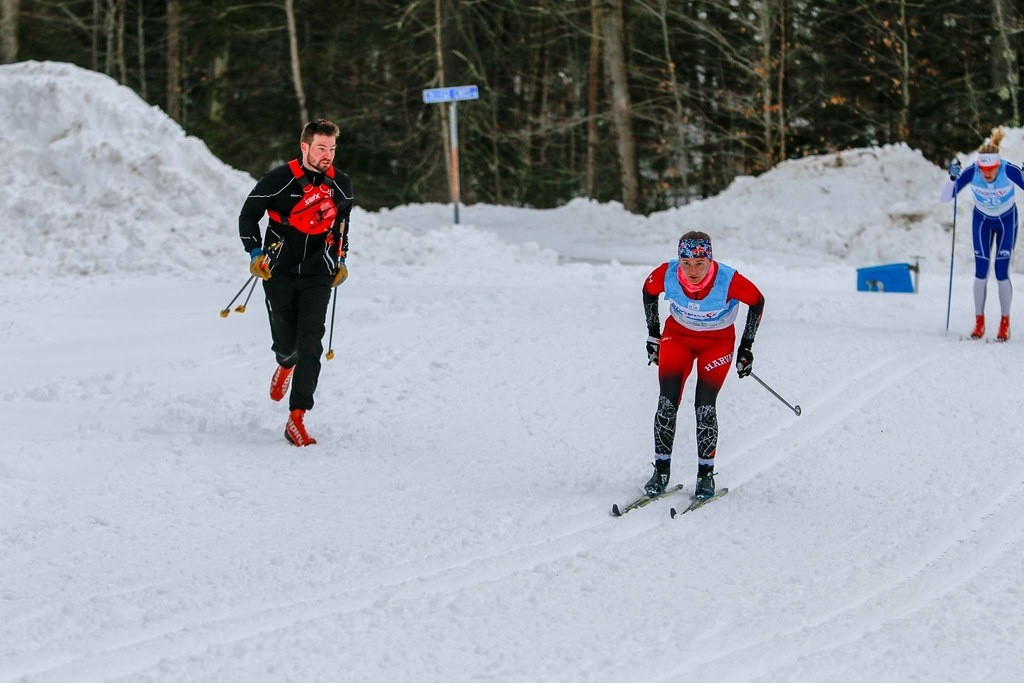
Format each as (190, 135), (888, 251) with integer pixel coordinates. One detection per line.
(331, 257), (348, 287)
(249, 247), (272, 281)
(646, 335), (662, 366)
(948, 159), (961, 180)
(737, 349), (753, 379)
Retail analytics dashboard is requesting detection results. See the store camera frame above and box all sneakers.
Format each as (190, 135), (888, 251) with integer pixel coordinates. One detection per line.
(270, 366), (295, 401)
(284, 415), (316, 447)
(996, 316), (1009, 340)
(695, 464), (718, 500)
(970, 315), (985, 339)
(645, 462), (669, 498)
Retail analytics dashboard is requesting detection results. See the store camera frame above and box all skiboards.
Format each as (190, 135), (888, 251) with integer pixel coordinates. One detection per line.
(958, 334), (1006, 344)
(612, 474), (730, 523)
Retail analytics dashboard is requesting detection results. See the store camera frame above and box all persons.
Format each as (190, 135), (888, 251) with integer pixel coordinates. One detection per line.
(642, 231), (765, 502)
(940, 125), (1024, 342)
(239, 119), (353, 447)
